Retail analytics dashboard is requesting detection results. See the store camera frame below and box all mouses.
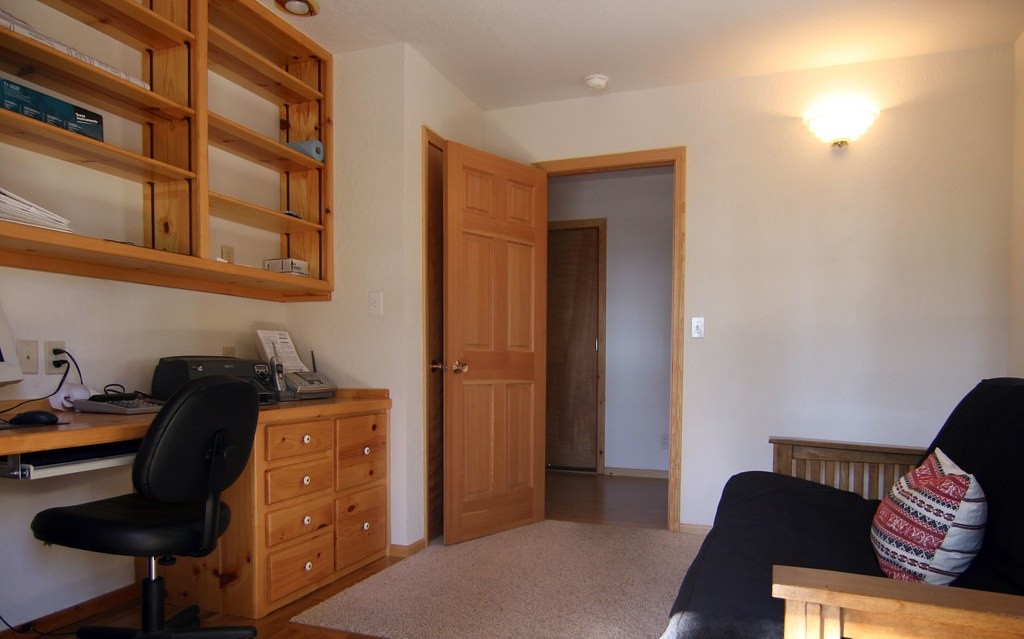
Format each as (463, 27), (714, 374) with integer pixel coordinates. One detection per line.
(8, 410), (59, 425)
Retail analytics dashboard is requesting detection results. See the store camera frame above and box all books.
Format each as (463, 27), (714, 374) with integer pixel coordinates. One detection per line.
(0, 187), (74, 234)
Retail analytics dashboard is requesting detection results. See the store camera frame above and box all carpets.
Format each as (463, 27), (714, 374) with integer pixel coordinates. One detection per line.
(289, 518), (707, 639)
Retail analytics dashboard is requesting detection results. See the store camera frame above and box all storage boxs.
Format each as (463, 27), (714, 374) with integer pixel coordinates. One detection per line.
(264, 259), (309, 275)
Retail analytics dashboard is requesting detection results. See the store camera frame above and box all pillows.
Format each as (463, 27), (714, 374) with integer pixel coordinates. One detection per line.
(868, 450), (987, 586)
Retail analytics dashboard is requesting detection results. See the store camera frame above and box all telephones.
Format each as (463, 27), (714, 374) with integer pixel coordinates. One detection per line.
(270, 341), (337, 402)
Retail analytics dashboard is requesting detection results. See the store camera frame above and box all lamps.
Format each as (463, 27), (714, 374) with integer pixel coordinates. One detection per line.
(800, 93), (881, 151)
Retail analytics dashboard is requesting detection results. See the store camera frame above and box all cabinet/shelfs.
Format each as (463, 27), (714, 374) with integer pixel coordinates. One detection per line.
(0, 390), (392, 639)
(0, 0), (334, 303)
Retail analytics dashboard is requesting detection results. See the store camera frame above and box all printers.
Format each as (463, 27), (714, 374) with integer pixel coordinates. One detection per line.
(151, 356), (280, 405)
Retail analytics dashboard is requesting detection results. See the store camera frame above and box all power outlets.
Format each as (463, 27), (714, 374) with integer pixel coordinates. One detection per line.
(45, 341), (65, 375)
(222, 246), (235, 263)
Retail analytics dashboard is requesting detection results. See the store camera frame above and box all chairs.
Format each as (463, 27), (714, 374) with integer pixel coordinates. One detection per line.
(29, 375), (259, 639)
(659, 377), (1024, 639)
